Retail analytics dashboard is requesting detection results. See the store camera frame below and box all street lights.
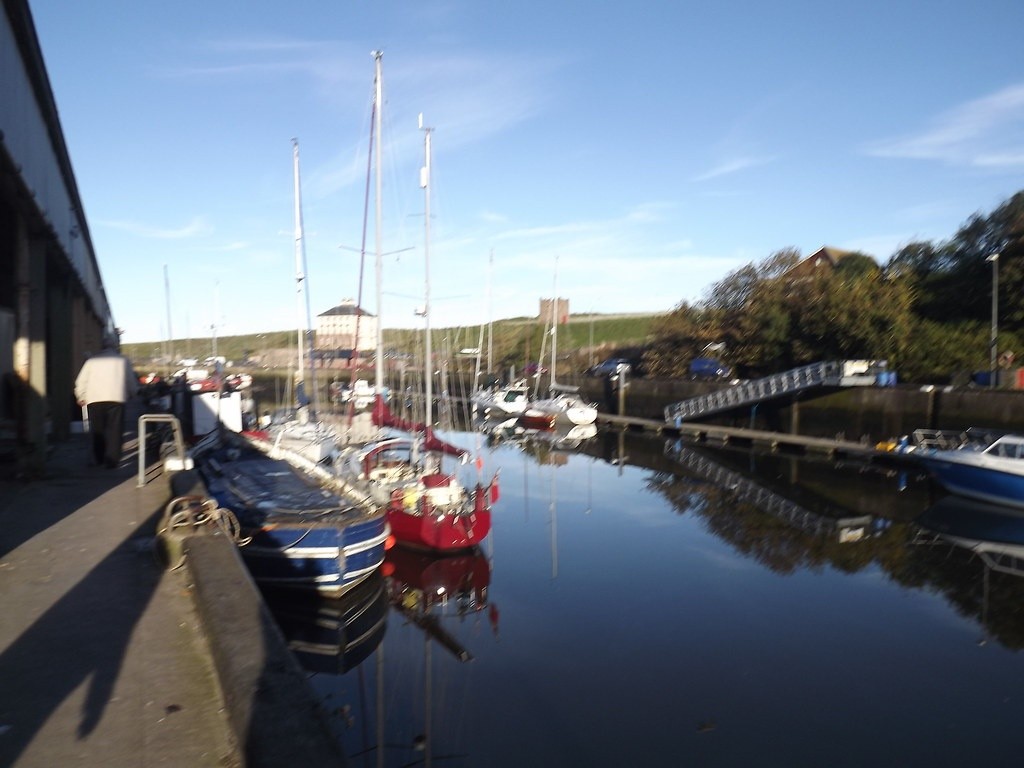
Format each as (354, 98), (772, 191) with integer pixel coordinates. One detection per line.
(986, 242), (1008, 387)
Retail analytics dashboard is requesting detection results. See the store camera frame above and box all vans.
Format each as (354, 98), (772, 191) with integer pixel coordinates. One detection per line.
(176, 358), (199, 367)
(203, 356), (226, 366)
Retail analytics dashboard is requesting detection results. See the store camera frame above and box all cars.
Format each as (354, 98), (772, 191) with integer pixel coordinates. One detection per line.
(521, 363), (548, 375)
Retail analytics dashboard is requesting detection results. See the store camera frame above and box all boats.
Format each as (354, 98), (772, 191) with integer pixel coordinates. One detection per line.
(471, 255), (598, 429)
(871, 426), (1024, 508)
(156, 53), (502, 600)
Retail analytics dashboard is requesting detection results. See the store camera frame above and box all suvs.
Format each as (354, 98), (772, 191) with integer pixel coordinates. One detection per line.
(589, 358), (632, 377)
(688, 358), (733, 383)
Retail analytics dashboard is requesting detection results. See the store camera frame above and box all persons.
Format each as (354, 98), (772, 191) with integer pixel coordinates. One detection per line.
(876, 361), (896, 387)
(74, 336), (138, 469)
